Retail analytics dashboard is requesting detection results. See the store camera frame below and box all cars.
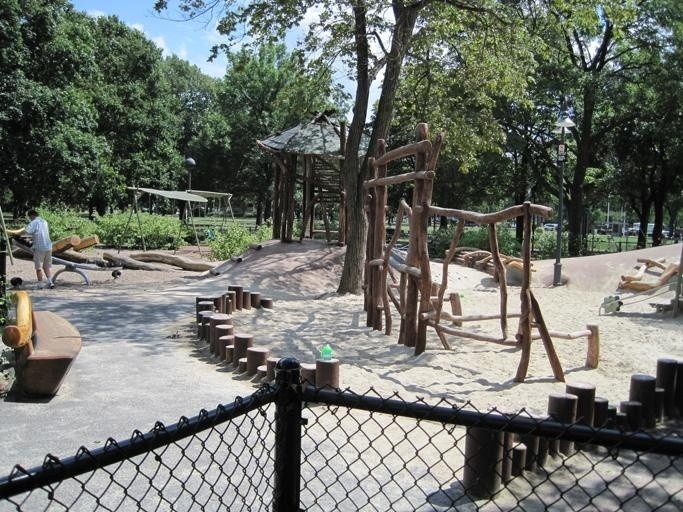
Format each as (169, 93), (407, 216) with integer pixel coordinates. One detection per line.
(541, 218), (678, 241)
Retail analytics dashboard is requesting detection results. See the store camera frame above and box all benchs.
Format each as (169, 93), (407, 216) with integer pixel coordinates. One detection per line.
(1, 290), (82, 399)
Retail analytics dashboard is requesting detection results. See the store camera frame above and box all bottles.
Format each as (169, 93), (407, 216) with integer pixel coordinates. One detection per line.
(320, 343), (333, 360)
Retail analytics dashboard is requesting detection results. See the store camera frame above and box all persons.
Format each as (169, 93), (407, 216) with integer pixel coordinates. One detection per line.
(12, 208), (55, 289)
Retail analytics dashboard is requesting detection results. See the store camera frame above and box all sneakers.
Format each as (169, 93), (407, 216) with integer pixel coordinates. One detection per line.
(48, 284), (54, 289)
(33, 286), (43, 290)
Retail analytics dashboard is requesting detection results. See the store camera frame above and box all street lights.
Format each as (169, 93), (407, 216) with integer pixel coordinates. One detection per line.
(184, 156), (198, 225)
(549, 93), (580, 287)
